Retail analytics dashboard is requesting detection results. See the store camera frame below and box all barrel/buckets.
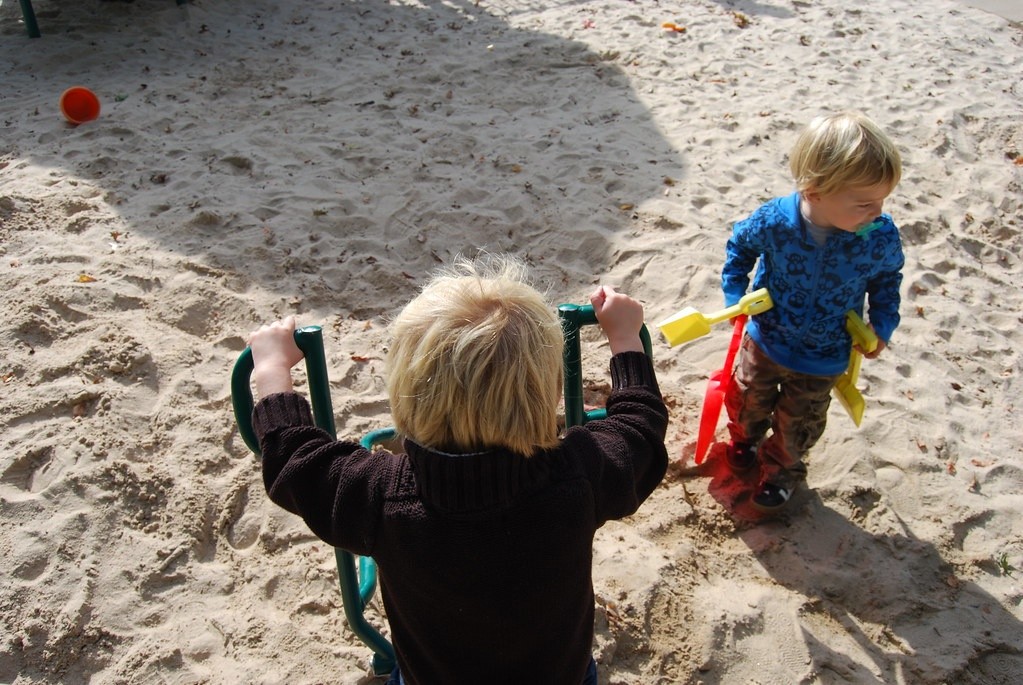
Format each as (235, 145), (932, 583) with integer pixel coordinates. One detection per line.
(58, 86), (100, 125)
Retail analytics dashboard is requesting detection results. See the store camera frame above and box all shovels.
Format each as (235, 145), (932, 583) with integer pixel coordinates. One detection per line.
(834, 310), (878, 427)
(655, 287), (774, 347)
(695, 315), (747, 464)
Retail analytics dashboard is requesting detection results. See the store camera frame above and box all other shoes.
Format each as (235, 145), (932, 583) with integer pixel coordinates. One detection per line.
(727, 438), (760, 476)
(751, 480), (795, 513)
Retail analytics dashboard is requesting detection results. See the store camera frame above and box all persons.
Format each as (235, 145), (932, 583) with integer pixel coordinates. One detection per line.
(721, 112), (904, 522)
(249, 267), (670, 685)
